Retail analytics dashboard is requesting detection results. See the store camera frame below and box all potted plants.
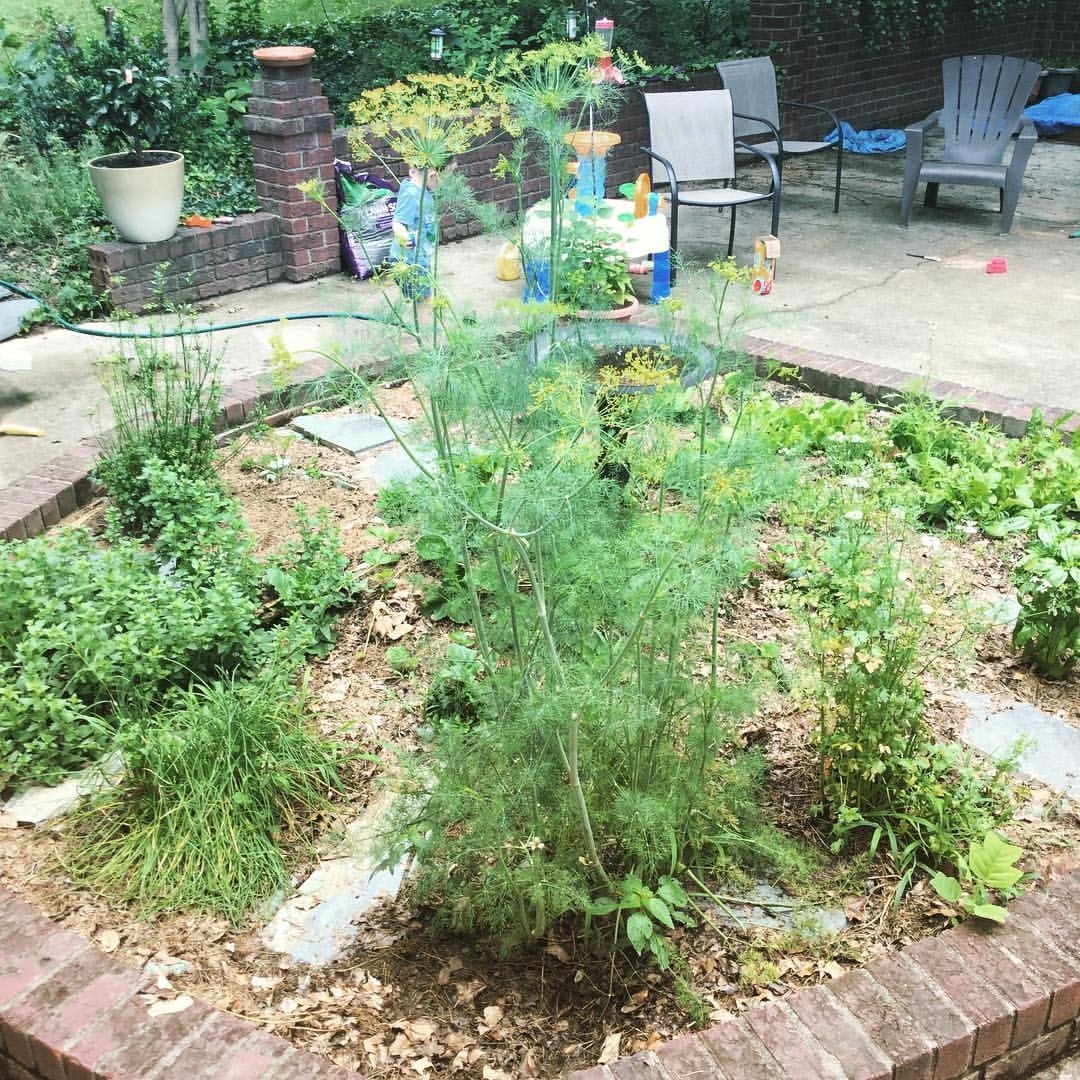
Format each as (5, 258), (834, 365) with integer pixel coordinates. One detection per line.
(548, 227), (638, 328)
(86, 67), (186, 244)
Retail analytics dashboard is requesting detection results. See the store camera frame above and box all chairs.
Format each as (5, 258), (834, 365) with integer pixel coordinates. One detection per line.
(643, 90), (783, 289)
(900, 55), (1042, 235)
(715, 56), (844, 235)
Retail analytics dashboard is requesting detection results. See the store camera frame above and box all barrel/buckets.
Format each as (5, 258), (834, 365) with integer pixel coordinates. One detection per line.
(496, 235), (524, 279)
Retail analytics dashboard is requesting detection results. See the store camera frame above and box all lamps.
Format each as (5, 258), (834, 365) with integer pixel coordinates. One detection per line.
(565, 7), (578, 39)
(430, 28), (446, 61)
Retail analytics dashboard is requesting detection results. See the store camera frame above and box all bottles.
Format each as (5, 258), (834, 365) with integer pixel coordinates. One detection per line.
(628, 264), (649, 274)
